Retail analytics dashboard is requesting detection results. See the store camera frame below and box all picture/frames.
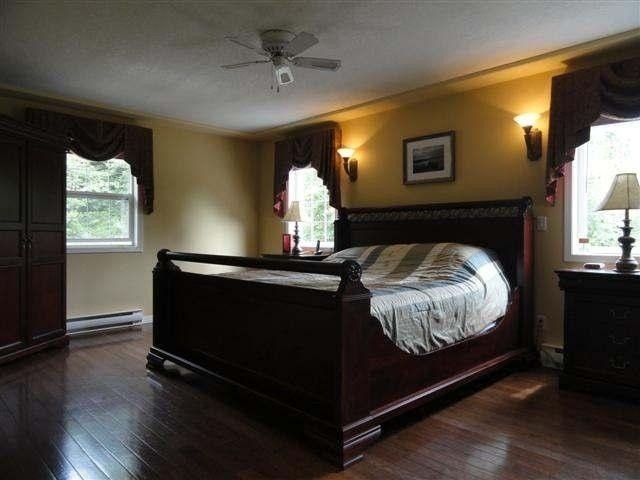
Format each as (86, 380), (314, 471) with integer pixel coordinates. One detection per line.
(402, 131), (455, 185)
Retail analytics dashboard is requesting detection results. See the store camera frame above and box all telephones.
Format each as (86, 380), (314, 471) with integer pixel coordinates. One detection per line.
(314, 240), (323, 255)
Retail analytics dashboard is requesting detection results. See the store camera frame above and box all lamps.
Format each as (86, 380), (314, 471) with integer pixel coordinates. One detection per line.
(595, 174), (640, 271)
(281, 200), (306, 254)
(337, 148), (357, 182)
(260, 30), (296, 86)
(513, 114), (542, 160)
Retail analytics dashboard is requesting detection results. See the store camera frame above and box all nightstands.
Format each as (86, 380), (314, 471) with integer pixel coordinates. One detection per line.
(262, 251), (333, 260)
(555, 264), (639, 405)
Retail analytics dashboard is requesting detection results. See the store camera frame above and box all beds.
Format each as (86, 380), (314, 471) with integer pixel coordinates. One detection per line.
(147, 196), (541, 471)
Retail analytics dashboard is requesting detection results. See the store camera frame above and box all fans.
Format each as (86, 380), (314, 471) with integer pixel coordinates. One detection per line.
(220, 28), (342, 93)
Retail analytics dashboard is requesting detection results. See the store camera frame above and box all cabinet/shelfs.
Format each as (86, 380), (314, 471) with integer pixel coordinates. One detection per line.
(1, 114), (67, 364)
